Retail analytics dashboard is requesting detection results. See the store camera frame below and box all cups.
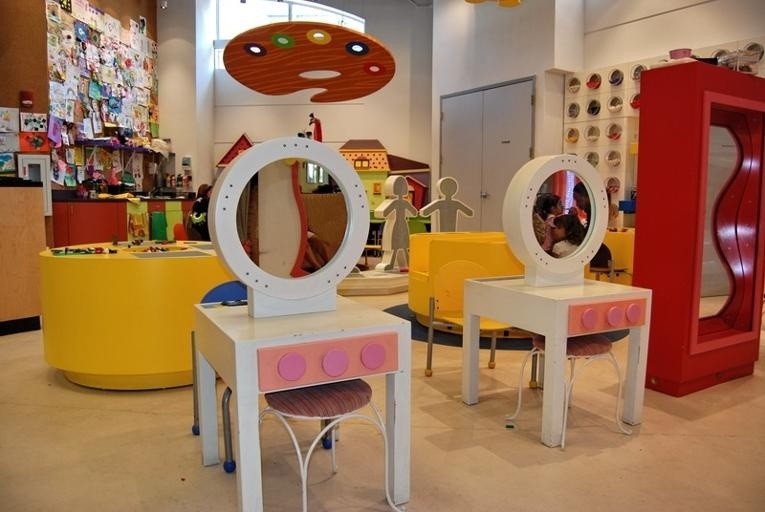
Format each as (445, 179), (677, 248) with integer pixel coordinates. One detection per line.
(165, 172), (188, 188)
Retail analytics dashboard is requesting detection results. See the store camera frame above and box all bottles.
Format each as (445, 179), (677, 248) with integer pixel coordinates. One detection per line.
(150, 204), (167, 239)
(82, 190), (96, 200)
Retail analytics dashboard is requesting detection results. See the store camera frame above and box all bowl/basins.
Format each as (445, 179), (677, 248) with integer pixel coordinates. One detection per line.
(52, 189), (81, 201)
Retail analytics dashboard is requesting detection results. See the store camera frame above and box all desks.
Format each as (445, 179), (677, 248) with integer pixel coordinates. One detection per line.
(191, 292), (413, 507)
(461, 275), (654, 448)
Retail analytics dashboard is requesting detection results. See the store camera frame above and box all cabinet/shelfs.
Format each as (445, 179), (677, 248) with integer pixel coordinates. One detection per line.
(52, 192), (199, 249)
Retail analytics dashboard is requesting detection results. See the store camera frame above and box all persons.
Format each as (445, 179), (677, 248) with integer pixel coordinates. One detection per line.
(186, 184), (213, 241)
(537, 181), (591, 258)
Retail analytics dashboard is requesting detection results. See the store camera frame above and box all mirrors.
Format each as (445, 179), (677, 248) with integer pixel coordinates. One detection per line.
(501, 154), (609, 284)
(207, 136), (371, 319)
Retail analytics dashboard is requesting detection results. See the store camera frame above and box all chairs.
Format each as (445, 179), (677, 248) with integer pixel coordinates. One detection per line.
(424, 260), (513, 377)
(406, 226), (636, 380)
(195, 280), (342, 472)
(590, 243), (629, 281)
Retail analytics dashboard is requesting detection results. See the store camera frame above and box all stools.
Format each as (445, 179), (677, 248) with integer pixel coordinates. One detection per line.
(504, 333), (633, 451)
(257, 377), (404, 512)
